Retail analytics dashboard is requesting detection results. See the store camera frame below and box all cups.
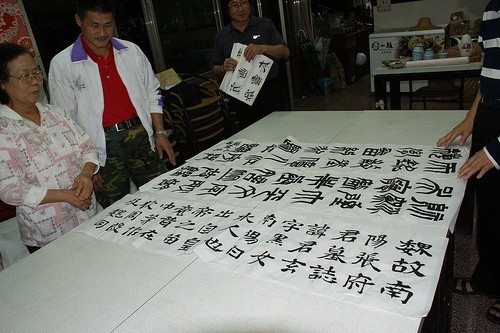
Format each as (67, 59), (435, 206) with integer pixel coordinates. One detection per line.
(440, 53), (448, 58)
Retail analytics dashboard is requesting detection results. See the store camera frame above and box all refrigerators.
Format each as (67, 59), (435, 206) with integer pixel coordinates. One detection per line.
(368, 23), (446, 93)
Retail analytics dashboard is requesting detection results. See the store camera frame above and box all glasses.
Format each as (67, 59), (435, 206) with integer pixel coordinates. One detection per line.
(8, 71), (46, 82)
(228, 2), (249, 10)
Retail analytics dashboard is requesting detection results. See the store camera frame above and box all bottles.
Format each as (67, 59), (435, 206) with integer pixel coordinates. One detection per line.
(412, 44), (423, 60)
(424, 48), (434, 60)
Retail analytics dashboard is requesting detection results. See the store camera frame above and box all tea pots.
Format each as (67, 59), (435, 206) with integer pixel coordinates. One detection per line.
(454, 34), (478, 56)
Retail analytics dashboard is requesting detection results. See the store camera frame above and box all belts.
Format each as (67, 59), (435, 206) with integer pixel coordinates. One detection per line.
(103, 117), (141, 133)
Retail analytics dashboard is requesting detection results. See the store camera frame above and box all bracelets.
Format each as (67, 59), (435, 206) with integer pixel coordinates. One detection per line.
(155, 130), (169, 138)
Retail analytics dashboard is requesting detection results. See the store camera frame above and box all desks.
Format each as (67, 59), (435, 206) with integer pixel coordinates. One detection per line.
(371, 57), (483, 110)
(0, 110), (474, 333)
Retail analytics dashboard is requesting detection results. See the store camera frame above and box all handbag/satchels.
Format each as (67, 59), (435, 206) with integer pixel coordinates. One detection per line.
(327, 52), (345, 90)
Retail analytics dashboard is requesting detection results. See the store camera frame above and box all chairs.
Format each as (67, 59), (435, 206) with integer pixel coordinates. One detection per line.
(152, 71), (230, 171)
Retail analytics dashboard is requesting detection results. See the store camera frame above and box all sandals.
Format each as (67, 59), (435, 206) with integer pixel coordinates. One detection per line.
(450, 277), (500, 299)
(485, 300), (500, 325)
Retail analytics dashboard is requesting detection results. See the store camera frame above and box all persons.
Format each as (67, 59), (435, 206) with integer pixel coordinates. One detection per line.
(209, 0), (290, 128)
(436, 0), (500, 323)
(49, 0), (176, 209)
(0, 42), (100, 255)
